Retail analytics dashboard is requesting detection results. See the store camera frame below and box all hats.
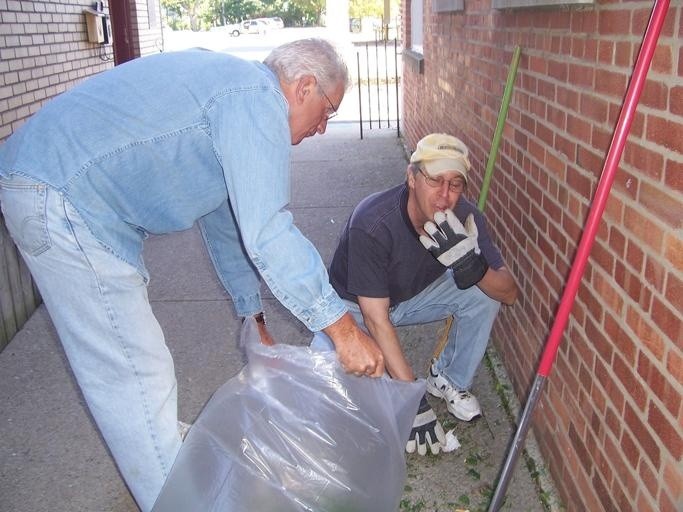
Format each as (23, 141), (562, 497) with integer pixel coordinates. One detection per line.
(409, 132), (471, 184)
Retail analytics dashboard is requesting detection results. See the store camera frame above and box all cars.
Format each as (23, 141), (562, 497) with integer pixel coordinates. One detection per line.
(255, 18), (285, 31)
(223, 19), (266, 37)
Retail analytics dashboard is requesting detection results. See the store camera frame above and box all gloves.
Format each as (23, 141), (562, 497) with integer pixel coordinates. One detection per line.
(424, 358), (482, 422)
(405, 378), (447, 456)
(419, 207), (490, 290)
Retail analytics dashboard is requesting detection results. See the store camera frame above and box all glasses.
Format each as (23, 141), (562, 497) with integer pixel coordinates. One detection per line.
(418, 168), (467, 194)
(315, 79), (339, 120)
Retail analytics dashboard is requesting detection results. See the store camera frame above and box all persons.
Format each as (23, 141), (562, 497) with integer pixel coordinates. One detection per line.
(310, 131), (520, 457)
(0, 35), (388, 511)
